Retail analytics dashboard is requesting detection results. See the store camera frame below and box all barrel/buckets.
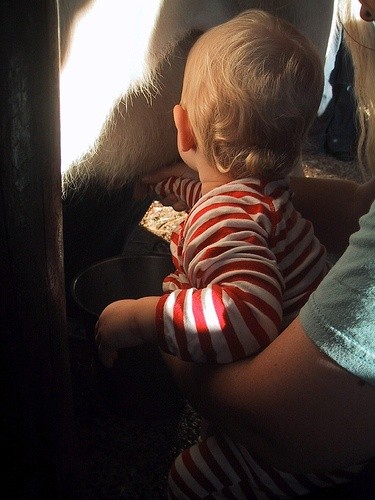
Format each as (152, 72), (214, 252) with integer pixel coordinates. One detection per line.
(72, 252), (186, 420)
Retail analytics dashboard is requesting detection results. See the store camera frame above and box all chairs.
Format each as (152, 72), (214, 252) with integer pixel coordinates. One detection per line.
(70, 172), (374, 354)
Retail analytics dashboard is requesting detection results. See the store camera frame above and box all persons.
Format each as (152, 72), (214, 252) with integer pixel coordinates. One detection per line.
(157, 187), (375, 480)
(92, 10), (364, 500)
